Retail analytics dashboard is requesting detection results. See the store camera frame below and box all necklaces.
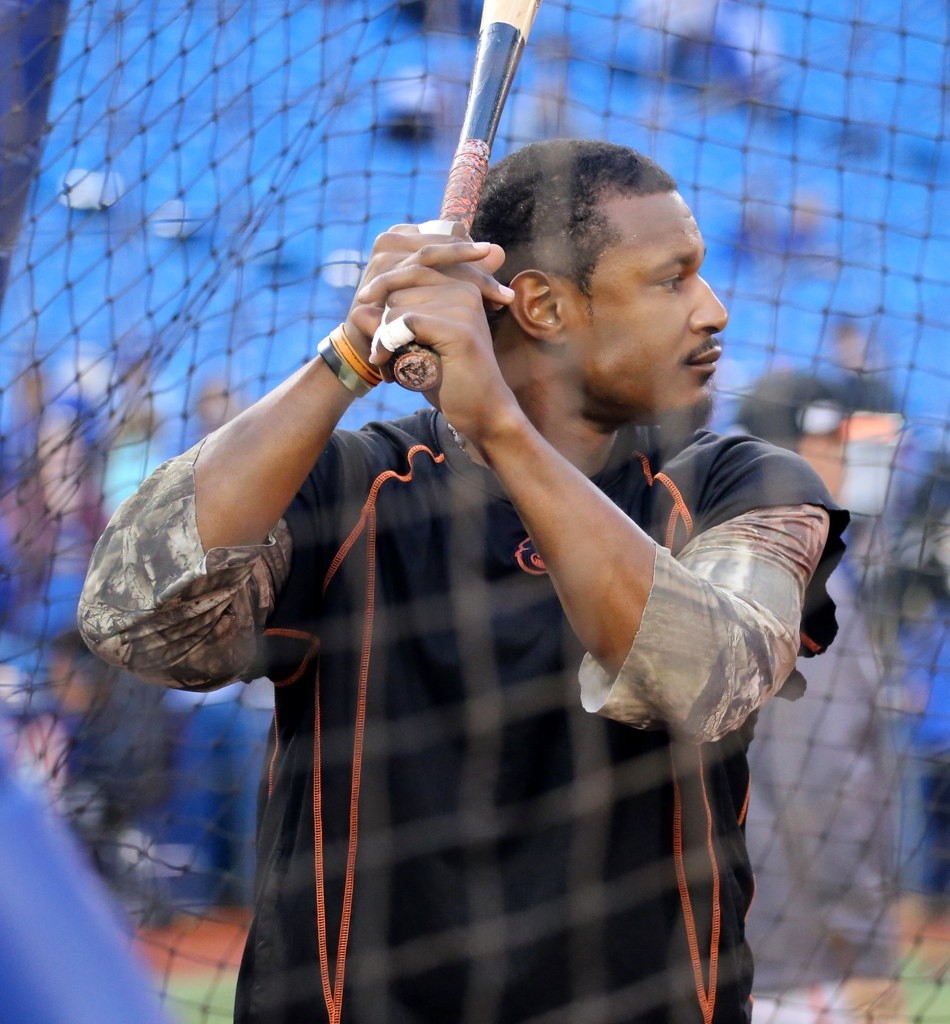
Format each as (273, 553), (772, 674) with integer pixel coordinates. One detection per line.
(447, 419), (466, 453)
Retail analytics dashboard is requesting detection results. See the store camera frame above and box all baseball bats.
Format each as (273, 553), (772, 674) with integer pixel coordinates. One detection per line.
(388, 0), (543, 390)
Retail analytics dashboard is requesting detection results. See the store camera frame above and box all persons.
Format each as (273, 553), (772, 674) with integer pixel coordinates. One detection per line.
(76, 137), (852, 1024)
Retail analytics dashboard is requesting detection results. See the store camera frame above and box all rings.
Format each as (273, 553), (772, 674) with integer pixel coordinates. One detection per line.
(378, 314), (416, 354)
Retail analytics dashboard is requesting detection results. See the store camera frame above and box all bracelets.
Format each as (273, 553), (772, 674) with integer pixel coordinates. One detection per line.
(317, 323), (383, 398)
